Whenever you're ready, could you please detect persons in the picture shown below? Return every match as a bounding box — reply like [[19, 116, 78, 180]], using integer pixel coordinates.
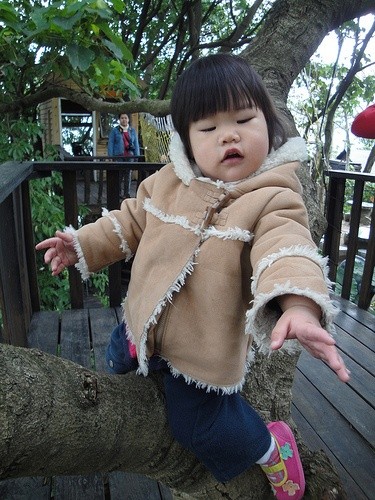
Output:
[[35, 53, 351, 500], [108, 113, 140, 199]]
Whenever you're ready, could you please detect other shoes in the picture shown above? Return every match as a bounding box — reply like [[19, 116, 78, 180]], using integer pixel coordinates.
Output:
[[260, 421, 305, 500]]
